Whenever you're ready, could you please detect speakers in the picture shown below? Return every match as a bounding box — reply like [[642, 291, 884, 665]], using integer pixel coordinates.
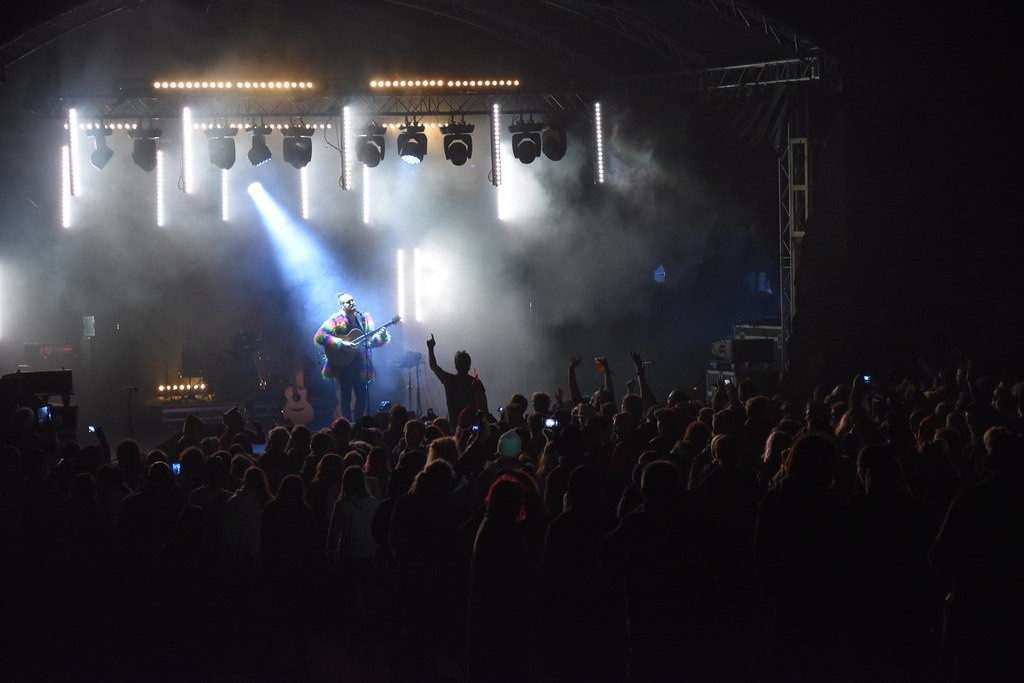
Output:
[[78, 333, 142, 430], [234, 331, 274, 404], [181, 346, 203, 377]]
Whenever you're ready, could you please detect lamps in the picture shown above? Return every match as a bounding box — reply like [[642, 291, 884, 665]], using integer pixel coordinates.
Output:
[[127, 118, 162, 172], [439, 114, 475, 166], [281, 115, 315, 170], [111, 79, 128, 96], [203, 116, 238, 170], [86, 118, 114, 171], [509, 110, 567, 164], [246, 117, 272, 166], [353, 116, 387, 167], [397, 114, 428, 164]]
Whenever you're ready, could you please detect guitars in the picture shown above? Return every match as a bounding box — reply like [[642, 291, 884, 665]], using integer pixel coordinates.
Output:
[[281, 346, 315, 425], [324, 314, 402, 368]]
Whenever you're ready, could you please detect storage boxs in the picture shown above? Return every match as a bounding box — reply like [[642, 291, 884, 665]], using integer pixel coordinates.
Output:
[[706, 370, 735, 405]]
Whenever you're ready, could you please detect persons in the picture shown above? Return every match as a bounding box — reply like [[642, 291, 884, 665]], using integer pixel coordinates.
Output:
[[0, 333, 1024, 682], [313, 293, 391, 422]]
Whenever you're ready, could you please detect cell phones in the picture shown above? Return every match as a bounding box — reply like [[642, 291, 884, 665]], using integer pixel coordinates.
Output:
[[88, 426, 97, 433], [864, 375, 872, 384], [542, 418, 559, 429], [472, 425, 480, 433], [172, 464, 181, 475]]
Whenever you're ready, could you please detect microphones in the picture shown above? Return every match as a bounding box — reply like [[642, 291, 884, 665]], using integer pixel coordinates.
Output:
[[353, 307, 364, 316]]
